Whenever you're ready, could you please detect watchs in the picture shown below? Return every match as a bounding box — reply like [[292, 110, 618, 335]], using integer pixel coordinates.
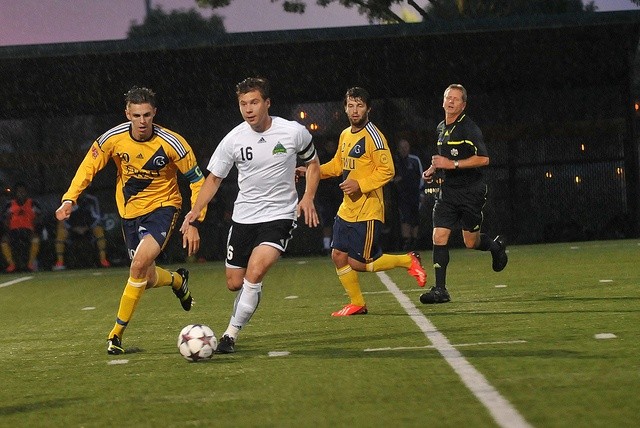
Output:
[[455, 159, 460, 170]]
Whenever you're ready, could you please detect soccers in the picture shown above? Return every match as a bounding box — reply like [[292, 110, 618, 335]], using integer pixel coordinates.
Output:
[[177, 322, 218, 363]]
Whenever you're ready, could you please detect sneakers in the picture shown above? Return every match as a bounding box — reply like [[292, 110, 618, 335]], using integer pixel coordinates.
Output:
[[331, 303, 367, 316], [406, 251, 427, 287], [491, 233, 508, 272], [172, 267, 193, 311], [419, 285, 451, 304], [105, 333, 126, 355], [218, 334, 236, 353]]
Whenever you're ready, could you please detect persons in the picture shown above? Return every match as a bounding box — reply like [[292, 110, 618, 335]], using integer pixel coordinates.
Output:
[[319, 139, 344, 257], [51, 189, 112, 271], [296, 86, 427, 317], [179, 76, 321, 357], [0, 181, 48, 274], [420, 82, 508, 305], [57, 85, 208, 358], [394, 139, 424, 251]]
